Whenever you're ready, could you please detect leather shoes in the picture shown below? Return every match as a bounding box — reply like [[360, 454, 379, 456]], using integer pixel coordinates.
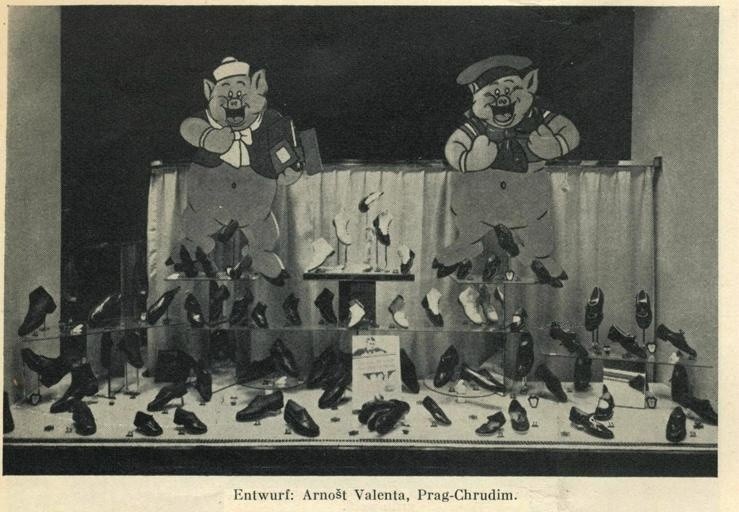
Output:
[[332, 191, 394, 247]]
